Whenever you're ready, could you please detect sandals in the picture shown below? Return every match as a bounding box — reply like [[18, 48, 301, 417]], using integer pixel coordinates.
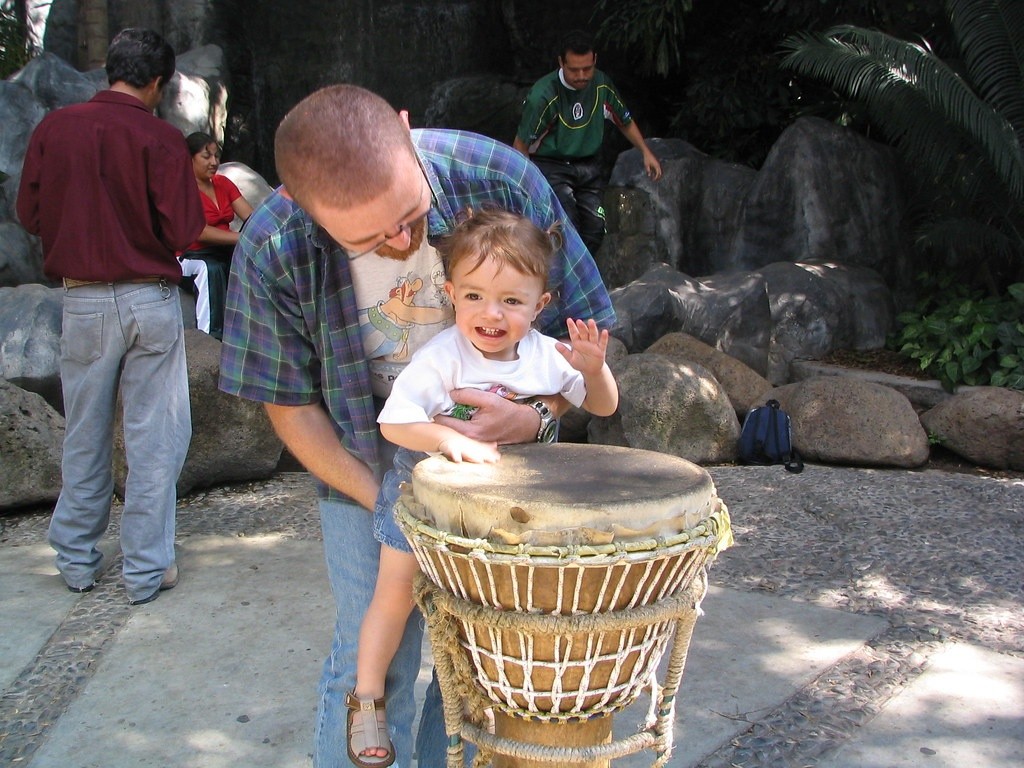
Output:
[[346, 687, 396, 768]]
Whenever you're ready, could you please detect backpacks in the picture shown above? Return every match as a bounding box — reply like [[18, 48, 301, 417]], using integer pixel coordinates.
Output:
[[737, 399, 804, 473]]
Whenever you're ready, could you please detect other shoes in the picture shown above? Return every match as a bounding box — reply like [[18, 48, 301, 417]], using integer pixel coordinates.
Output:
[[132, 563, 180, 607]]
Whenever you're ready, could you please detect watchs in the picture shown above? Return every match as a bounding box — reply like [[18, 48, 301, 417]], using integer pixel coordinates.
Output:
[[523, 397, 558, 444]]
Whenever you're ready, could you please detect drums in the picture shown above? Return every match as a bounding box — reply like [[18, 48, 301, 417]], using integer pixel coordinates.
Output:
[[386, 440, 746, 768]]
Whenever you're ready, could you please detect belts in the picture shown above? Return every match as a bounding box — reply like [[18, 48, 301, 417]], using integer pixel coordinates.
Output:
[[62, 274, 166, 290]]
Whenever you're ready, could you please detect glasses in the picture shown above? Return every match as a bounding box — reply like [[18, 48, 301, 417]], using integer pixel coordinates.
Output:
[[324, 151, 440, 262]]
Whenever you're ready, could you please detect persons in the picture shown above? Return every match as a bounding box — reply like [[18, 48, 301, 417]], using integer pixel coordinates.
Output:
[[168, 131, 255, 342], [14, 27, 209, 607], [215, 82, 619, 767], [512, 28, 662, 259]]
